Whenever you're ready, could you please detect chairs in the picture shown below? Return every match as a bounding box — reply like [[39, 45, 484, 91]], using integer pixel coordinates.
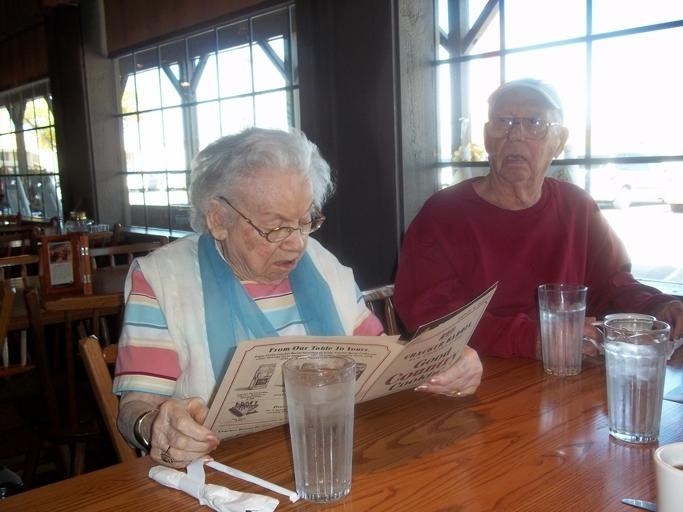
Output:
[[1, 210, 406, 463]]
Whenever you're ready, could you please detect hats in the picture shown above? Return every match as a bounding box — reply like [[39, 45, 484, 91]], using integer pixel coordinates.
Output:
[[489, 77, 560, 111]]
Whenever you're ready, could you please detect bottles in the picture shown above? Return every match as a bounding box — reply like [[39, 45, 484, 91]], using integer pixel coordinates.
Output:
[[63, 216, 93, 236]]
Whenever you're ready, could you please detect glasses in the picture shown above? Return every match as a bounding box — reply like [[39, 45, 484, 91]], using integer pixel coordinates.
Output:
[[219, 196, 326, 244], [488, 118, 560, 139]]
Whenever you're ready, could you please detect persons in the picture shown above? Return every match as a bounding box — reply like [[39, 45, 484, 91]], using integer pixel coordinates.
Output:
[[392, 78, 682, 362], [112, 126, 484, 471]]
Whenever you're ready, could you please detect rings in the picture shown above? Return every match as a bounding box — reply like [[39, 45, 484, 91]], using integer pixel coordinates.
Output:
[[452, 390, 462, 399], [160, 445, 173, 463]]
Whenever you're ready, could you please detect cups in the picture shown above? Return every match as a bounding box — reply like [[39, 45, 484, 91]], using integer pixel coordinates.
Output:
[[537, 283, 683, 512], [283, 354, 357, 503]]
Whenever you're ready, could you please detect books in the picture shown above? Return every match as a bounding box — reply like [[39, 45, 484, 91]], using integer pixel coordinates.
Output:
[[202, 279, 500, 443]]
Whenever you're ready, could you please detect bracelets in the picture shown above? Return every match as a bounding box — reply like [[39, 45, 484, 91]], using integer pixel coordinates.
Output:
[[132, 409, 159, 451]]
